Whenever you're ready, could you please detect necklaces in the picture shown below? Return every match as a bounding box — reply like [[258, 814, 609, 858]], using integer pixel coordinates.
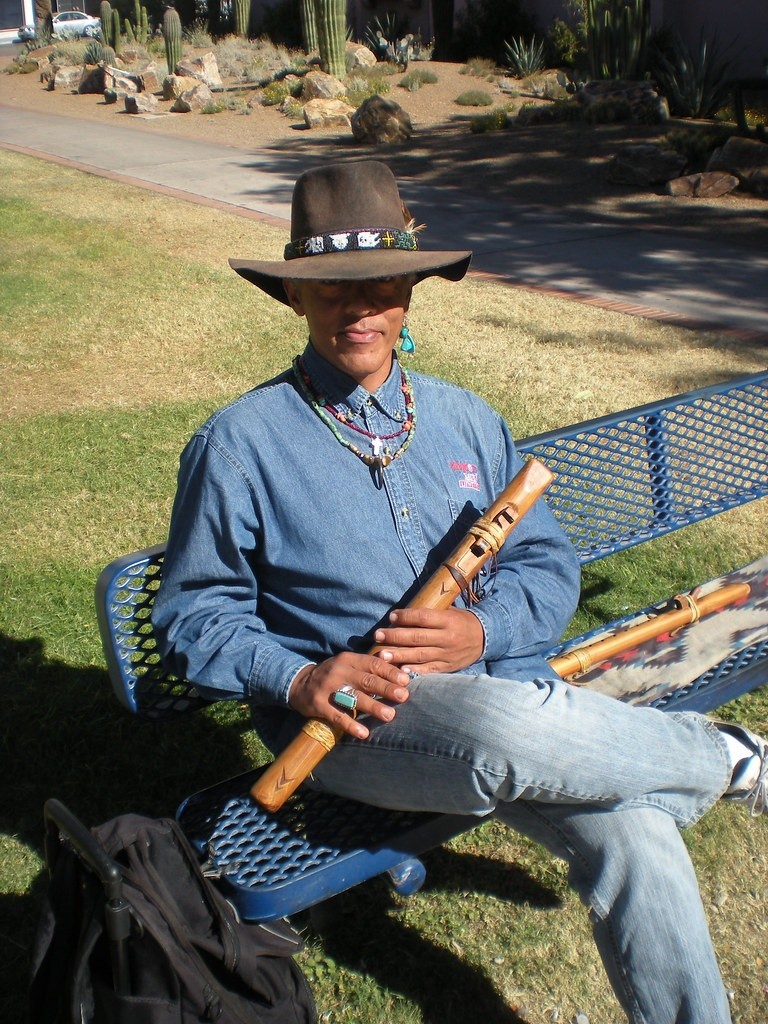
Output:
[[299, 354, 413, 456], [292, 354, 417, 487]]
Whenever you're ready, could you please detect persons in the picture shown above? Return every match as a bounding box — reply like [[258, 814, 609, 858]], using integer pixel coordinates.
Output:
[[151, 162, 768, 1024]]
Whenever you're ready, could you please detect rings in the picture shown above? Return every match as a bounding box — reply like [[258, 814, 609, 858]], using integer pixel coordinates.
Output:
[[332, 685, 357, 710]]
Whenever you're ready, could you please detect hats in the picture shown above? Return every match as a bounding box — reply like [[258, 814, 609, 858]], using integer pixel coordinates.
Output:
[[227, 160, 473, 308]]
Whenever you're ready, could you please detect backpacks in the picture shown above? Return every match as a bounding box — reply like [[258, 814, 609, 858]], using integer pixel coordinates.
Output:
[[24, 796, 318, 1024]]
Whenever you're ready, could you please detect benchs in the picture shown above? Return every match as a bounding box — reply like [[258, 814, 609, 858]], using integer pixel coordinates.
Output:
[[93, 371, 768, 930]]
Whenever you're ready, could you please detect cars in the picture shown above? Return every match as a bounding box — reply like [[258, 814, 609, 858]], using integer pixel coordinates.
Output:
[[17, 10, 101, 41]]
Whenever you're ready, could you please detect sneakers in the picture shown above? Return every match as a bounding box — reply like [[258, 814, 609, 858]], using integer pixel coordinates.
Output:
[[711, 719, 768, 818]]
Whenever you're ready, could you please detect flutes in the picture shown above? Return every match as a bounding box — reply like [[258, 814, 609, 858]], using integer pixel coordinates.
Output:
[[546, 583, 751, 679], [248, 458, 555, 814]]
